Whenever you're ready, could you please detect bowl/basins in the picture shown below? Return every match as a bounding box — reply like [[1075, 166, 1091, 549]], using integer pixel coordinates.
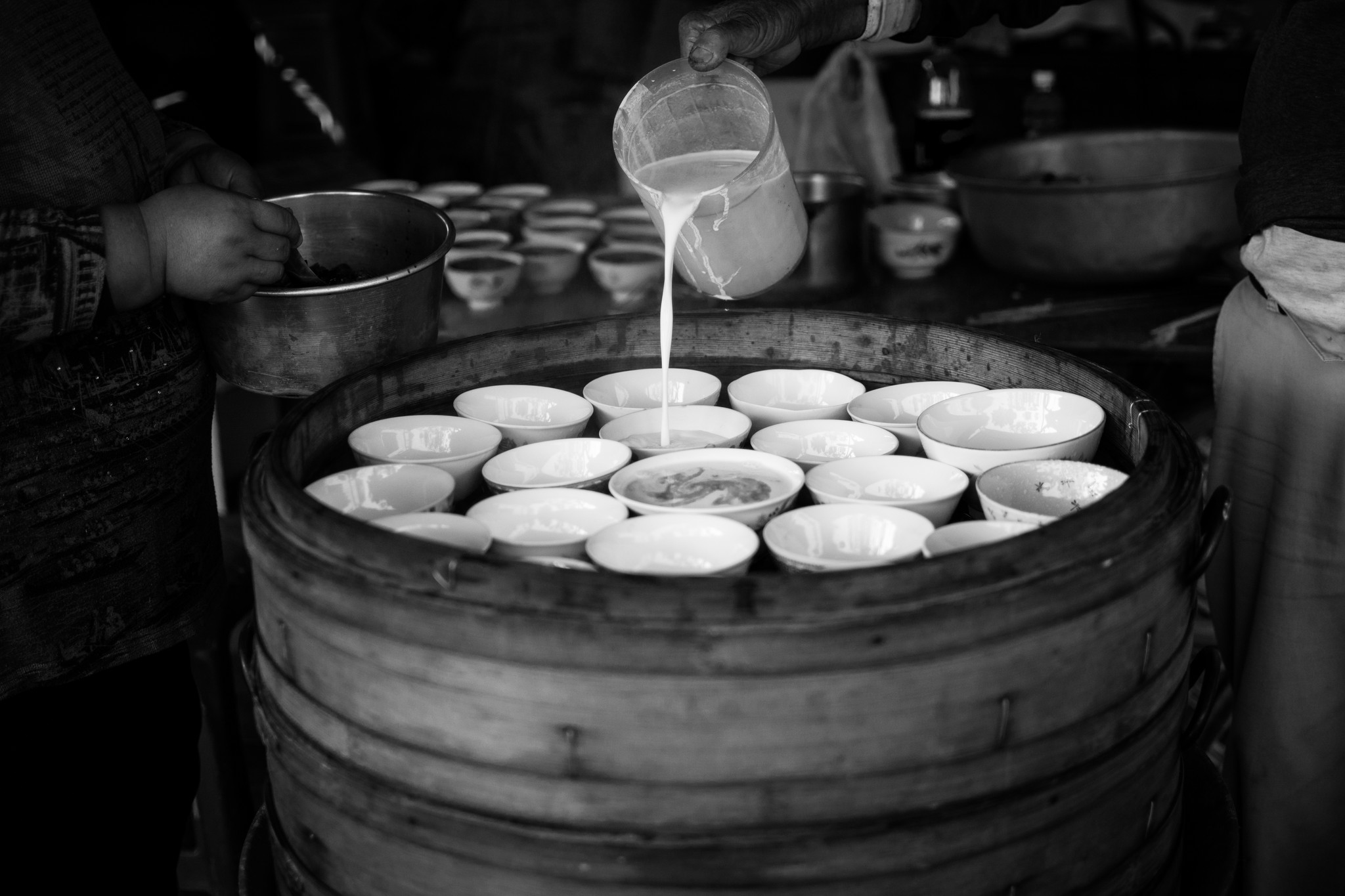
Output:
[[742, 165, 873, 296], [349, 161, 676, 316], [220, 175, 455, 401], [869, 199, 959, 279], [305, 355, 1127, 587], [948, 117, 1245, 279]]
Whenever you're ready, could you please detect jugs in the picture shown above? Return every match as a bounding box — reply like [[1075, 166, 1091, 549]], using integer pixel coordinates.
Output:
[[612, 52, 812, 304]]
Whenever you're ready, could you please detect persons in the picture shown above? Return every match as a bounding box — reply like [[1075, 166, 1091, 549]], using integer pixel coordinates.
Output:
[[678, 0, 1345, 895], [2, 3, 309, 896]]
